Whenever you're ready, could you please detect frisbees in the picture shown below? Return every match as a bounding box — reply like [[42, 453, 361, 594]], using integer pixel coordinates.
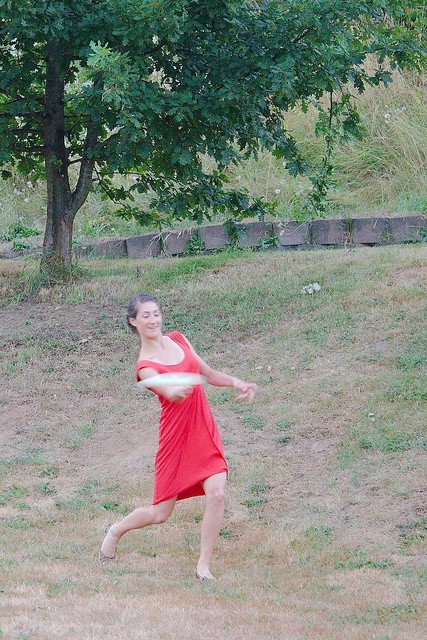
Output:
[[138, 373, 206, 387]]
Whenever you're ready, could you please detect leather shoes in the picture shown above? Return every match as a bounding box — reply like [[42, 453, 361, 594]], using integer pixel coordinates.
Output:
[[195, 565, 217, 581], [99, 546, 116, 566]]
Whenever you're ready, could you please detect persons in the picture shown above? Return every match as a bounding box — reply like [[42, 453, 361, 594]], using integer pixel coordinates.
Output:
[[100, 294, 257, 581]]
